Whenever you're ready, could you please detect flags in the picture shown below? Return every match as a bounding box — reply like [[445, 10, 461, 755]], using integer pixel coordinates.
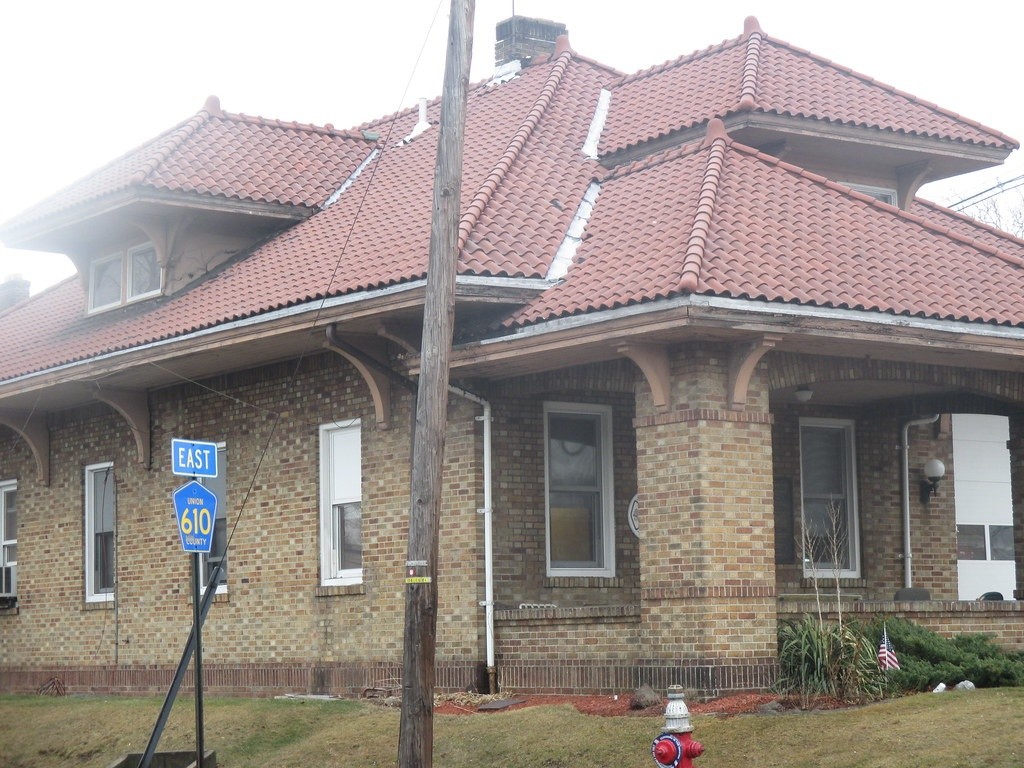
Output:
[[878, 627, 900, 672]]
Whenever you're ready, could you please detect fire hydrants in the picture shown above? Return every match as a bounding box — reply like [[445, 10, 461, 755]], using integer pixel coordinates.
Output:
[[653, 683, 704, 768]]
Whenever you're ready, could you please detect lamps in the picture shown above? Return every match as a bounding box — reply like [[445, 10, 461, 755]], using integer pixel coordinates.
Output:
[[920, 459, 945, 503], [795, 388, 814, 402]]
